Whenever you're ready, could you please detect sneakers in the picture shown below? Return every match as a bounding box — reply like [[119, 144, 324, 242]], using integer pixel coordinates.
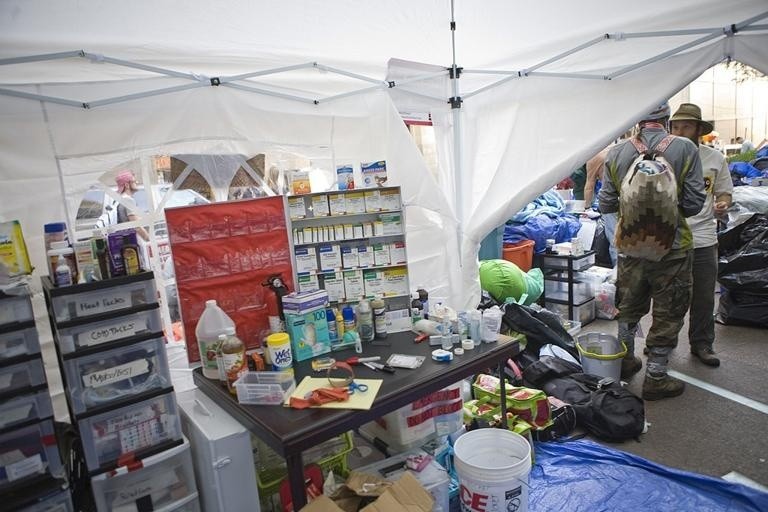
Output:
[[691, 342, 720, 366], [643, 374, 683, 400], [621, 356, 641, 377]]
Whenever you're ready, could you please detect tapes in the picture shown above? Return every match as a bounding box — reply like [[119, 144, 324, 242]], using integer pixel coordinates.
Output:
[[327, 362, 353, 387]]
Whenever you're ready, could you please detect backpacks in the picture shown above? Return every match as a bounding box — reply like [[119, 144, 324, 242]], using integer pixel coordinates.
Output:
[[615, 137, 678, 262]]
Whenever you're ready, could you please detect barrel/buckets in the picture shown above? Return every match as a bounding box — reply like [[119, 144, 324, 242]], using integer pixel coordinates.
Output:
[[195, 299, 237, 381], [453, 427, 532, 511]]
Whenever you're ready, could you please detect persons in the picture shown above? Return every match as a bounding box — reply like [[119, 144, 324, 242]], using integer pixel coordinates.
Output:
[[583, 141, 620, 268], [735, 136, 755, 155], [667, 102, 734, 368], [597, 100, 707, 402], [111, 169, 150, 244]]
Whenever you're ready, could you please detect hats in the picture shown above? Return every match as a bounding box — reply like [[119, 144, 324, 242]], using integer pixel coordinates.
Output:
[[670, 104, 713, 135], [115, 172, 134, 193], [644, 102, 670, 121]]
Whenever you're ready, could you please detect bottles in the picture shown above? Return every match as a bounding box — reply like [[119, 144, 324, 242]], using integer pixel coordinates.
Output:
[[291, 171, 311, 194], [410, 284, 429, 327], [326, 295, 387, 344], [43, 222, 78, 286], [337, 163, 354, 190], [442, 309, 501, 350], [214, 327, 249, 394], [96, 239, 115, 280], [267, 333, 293, 368], [120, 234, 141, 276]]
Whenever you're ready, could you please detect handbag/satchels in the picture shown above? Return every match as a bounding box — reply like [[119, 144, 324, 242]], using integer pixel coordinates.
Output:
[[463, 303, 644, 465]]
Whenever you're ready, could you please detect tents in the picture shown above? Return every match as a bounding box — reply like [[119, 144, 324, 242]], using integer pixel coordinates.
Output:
[[1, 1, 768, 511]]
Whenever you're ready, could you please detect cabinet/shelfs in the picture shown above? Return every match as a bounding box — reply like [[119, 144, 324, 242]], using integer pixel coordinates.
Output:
[[536, 250, 597, 328], [191, 329, 521, 512], [0, 274, 74, 512], [287, 186, 414, 335], [40, 269, 203, 512]]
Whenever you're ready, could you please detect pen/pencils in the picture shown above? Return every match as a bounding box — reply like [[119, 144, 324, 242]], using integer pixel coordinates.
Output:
[[363, 362, 375, 370], [346, 356, 381, 364], [368, 361, 395, 373]]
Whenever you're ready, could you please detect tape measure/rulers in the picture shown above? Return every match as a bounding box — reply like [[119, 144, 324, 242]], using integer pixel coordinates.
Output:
[[431, 349, 453, 362]]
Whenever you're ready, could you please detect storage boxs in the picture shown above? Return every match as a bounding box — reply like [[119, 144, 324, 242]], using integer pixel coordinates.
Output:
[[351, 447, 449, 512], [298, 471, 434, 511], [503, 239, 535, 273]]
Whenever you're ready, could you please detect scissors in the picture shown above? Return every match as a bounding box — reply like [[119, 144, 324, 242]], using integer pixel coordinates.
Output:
[[346, 380, 369, 395]]
